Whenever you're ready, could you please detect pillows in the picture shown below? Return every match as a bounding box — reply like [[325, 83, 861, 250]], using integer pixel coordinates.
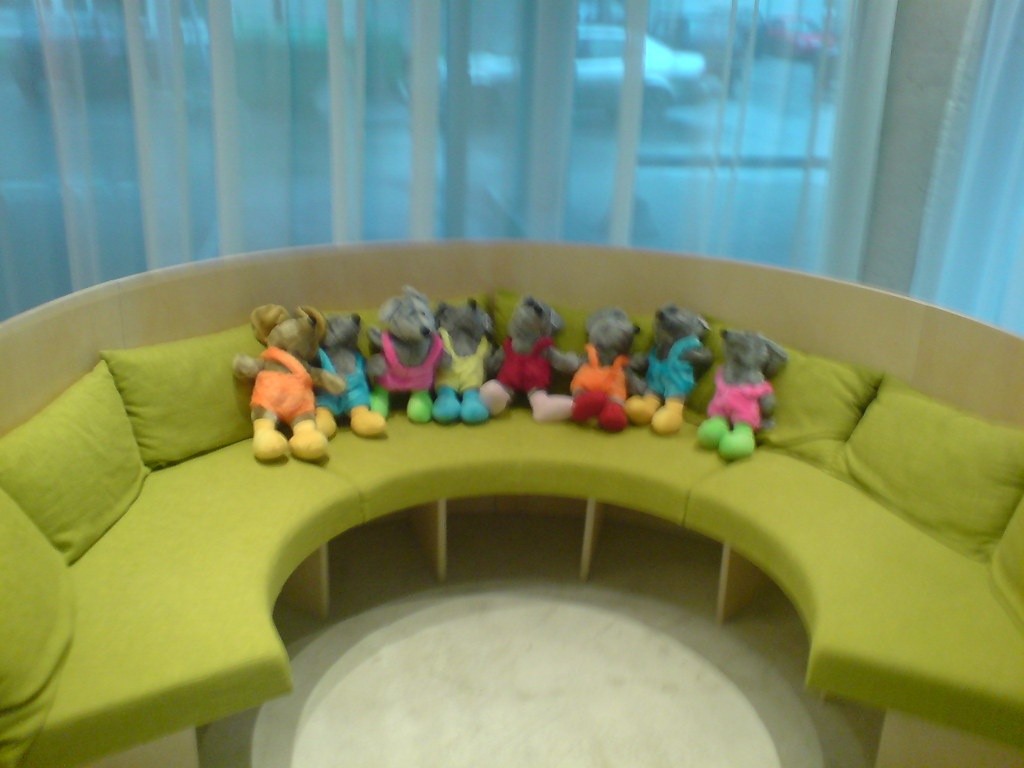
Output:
[[322, 291, 491, 387], [494, 285, 661, 392], [100, 322, 265, 470], [1, 360, 152, 565], [0, 487, 76, 768], [687, 320, 884, 465], [841, 373, 1022, 558]]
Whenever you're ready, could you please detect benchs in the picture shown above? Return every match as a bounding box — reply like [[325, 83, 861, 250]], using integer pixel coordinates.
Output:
[[0, 239, 1023, 768]]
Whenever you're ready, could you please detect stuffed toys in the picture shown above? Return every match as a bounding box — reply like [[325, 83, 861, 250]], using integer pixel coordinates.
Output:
[[571, 307, 646, 432], [623, 305, 715, 432], [369, 286, 497, 423], [697, 327, 787, 460], [480, 296, 580, 421], [234, 304, 388, 459]]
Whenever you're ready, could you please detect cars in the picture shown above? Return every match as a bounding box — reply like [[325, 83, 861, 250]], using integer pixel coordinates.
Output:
[[747, 12, 833, 58], [404, 9, 744, 127]]
